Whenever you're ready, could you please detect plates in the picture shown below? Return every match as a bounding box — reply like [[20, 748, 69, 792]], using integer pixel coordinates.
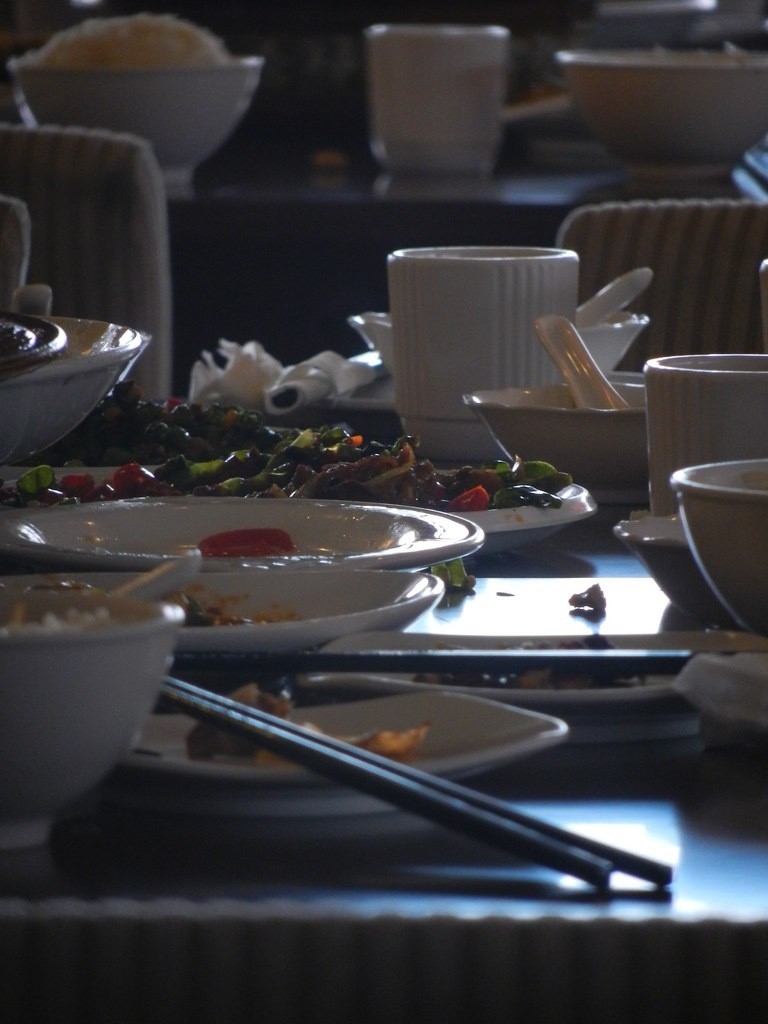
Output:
[[299, 631, 768, 719], [0, 498, 484, 570], [268, 366, 645, 435], [0, 571, 446, 657], [0, 466, 598, 558], [100, 691, 568, 816]]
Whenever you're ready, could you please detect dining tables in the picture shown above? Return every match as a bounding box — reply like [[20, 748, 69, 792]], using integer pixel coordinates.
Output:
[[0, 378, 768, 1024]]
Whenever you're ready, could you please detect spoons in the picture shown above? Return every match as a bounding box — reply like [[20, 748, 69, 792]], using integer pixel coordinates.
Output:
[[536, 314, 630, 408]]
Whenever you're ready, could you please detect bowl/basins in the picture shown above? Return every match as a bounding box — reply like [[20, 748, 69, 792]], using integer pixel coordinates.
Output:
[[0, 590, 185, 849], [671, 459, 768, 638], [466, 383, 651, 505], [0, 315, 150, 465], [7, 55, 264, 188], [347, 312, 649, 377], [614, 514, 737, 628], [556, 49, 768, 176]]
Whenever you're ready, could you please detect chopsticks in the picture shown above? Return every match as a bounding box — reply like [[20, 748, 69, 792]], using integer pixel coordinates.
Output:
[[163, 675, 672, 885], [171, 648, 690, 675]]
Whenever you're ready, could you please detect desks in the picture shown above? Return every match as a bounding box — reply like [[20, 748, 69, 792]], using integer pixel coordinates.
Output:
[[174, 165, 768, 378]]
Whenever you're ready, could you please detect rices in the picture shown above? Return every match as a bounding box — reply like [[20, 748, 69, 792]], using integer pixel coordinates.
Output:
[[15, 11, 241, 70]]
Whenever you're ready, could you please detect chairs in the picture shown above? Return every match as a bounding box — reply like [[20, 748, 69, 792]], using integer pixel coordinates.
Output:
[[1, 122, 176, 398], [558, 200, 768, 370]]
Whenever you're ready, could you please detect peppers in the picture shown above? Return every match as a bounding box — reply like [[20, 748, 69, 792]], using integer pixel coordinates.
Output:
[[1, 379, 571, 590]]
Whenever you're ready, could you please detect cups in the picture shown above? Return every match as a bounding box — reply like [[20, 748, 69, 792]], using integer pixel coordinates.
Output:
[[367, 23, 509, 177], [644, 353, 768, 517], [388, 246, 579, 459]]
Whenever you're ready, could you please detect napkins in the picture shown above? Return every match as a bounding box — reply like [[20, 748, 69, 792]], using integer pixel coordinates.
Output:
[[187, 343, 374, 413], [676, 651, 768, 740]]
